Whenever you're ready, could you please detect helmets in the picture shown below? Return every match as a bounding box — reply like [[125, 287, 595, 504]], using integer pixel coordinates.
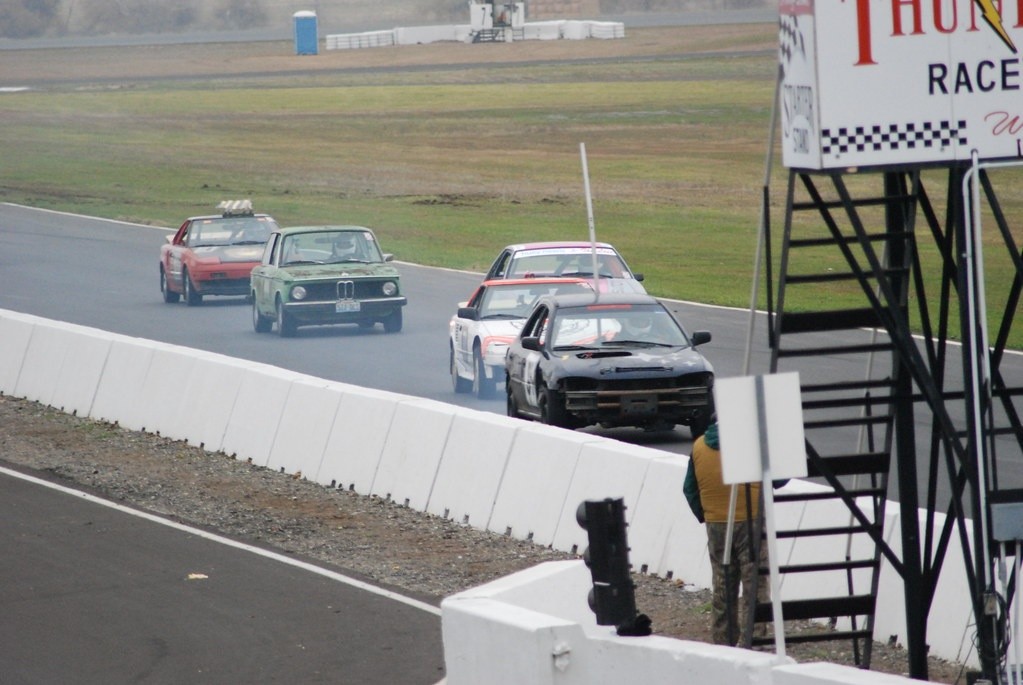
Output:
[[623, 315, 652, 336], [578, 255, 603, 274], [245, 222, 266, 242], [333, 234, 356, 257]]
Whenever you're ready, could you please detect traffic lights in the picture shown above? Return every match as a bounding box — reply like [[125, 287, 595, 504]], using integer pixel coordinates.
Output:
[[576, 496, 639, 628]]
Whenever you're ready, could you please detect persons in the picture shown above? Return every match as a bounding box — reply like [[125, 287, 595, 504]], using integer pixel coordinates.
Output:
[[331, 234, 368, 260], [614, 313, 661, 340], [683, 412, 792, 652], [576, 255, 604, 271]]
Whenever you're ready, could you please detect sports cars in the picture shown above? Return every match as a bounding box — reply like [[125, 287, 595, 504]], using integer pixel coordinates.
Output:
[[484, 238, 650, 309], [450, 276, 619, 402], [159, 199, 282, 308], [250, 224, 407, 338], [502, 290, 716, 438]]
